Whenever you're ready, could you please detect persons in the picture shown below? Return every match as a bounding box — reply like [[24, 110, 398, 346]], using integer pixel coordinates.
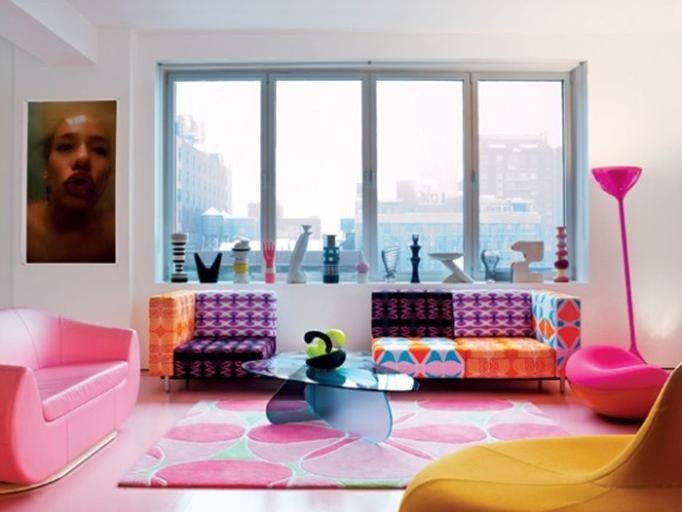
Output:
[[27, 113, 115, 261]]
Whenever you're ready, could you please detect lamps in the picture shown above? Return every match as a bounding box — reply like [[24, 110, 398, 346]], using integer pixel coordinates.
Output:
[[593, 164, 647, 360]]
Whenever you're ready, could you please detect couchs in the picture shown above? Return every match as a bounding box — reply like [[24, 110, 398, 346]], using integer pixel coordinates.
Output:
[[2, 306, 142, 486], [398, 362, 682, 511], [371, 289, 581, 393], [149, 289, 277, 393], [566, 345, 669, 420]]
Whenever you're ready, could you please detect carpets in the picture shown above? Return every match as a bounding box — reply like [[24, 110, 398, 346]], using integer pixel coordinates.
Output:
[[118, 398, 572, 489]]
